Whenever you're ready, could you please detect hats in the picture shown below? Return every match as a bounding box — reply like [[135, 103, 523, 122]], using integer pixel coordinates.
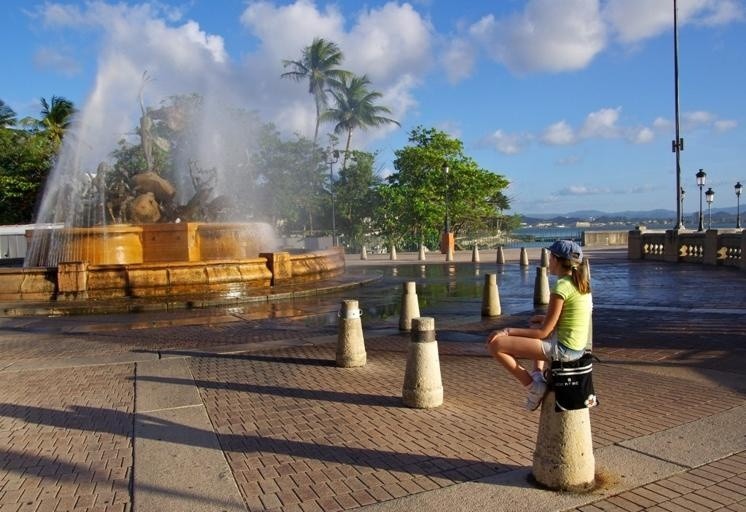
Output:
[[544, 239, 584, 260]]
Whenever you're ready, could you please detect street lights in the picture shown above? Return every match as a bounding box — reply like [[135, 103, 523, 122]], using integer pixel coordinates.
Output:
[[442, 162, 450, 233], [321, 150, 339, 246], [680, 169, 743, 232]]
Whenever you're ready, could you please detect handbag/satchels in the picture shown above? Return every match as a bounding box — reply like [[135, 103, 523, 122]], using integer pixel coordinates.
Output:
[[552, 355, 601, 413]]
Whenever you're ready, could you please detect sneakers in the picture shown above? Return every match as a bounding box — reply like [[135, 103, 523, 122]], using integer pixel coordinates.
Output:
[[532, 371, 546, 384], [526, 383, 547, 411]]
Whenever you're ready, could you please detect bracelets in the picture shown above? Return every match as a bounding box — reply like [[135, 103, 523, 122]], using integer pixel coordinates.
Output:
[[501, 327, 512, 336]]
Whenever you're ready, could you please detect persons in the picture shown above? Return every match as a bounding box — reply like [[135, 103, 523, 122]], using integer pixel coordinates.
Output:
[[136, 89, 162, 173], [485, 239, 593, 411]]
